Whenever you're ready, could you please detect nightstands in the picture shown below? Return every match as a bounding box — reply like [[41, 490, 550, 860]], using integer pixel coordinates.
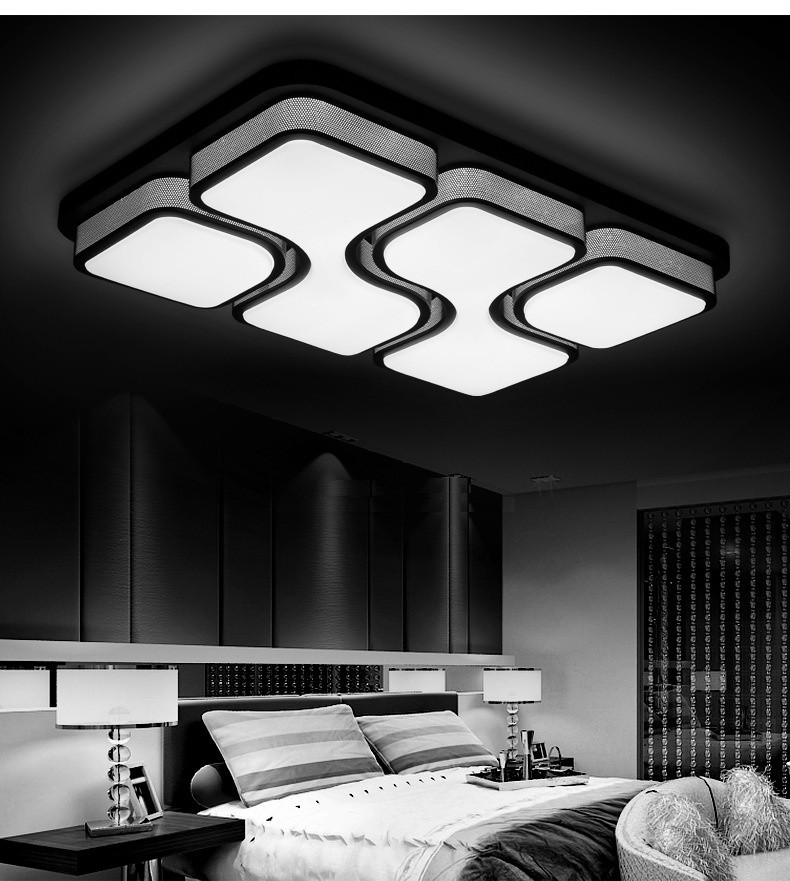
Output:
[[498, 754, 574, 772]]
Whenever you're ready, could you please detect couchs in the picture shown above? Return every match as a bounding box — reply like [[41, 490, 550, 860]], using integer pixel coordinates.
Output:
[[615, 775, 790, 881]]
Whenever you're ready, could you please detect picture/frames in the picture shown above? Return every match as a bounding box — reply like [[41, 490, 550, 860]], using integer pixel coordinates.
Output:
[[124, 762, 164, 821]]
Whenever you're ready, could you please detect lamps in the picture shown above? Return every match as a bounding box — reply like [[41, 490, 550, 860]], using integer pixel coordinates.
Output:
[[56, 30, 730, 397], [56, 669, 179, 837], [483, 667, 542, 760], [388, 667, 446, 691], [691, 671, 727, 692]]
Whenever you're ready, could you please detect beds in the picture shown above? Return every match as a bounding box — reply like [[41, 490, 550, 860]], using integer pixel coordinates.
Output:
[[643, 660, 790, 784], [164, 691, 666, 881]]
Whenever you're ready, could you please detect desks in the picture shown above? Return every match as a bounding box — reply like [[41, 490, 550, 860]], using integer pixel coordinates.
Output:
[[0, 811, 245, 881]]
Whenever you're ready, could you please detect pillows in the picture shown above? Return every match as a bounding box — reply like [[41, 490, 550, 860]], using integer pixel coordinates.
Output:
[[202, 704, 386, 808], [355, 710, 500, 775], [648, 688, 701, 728], [643, 702, 671, 738]]
